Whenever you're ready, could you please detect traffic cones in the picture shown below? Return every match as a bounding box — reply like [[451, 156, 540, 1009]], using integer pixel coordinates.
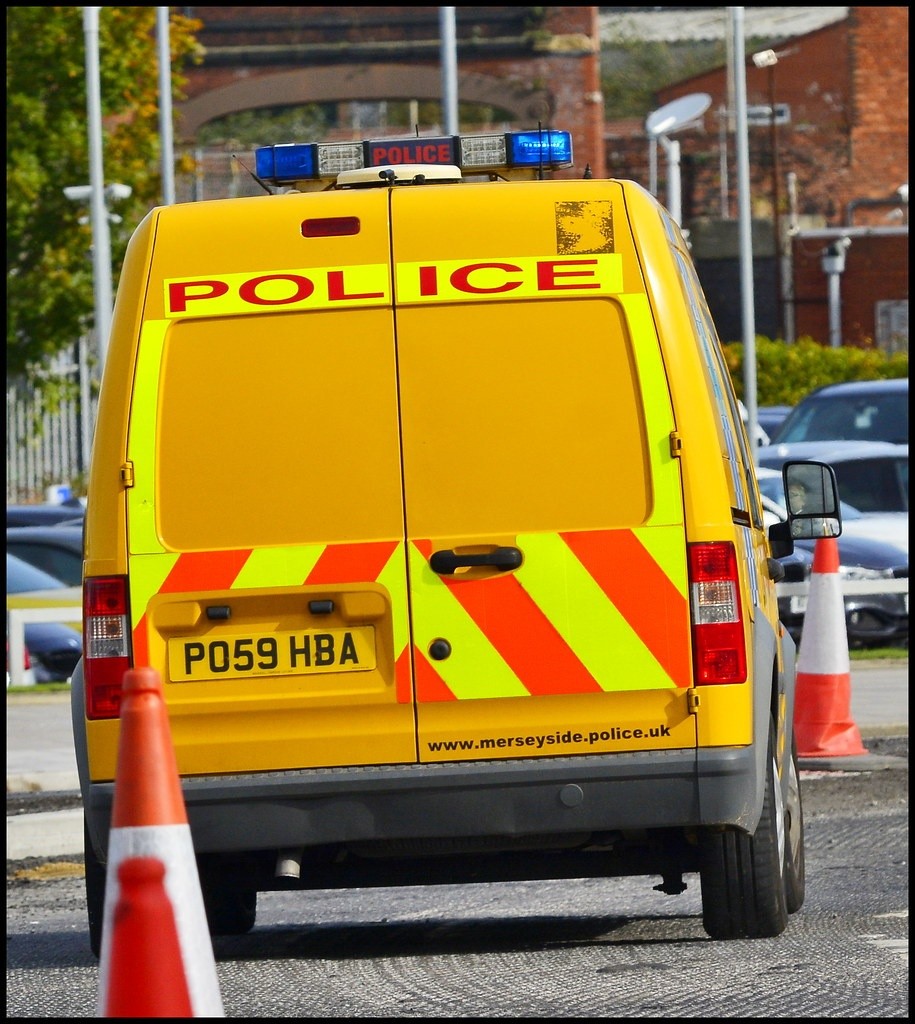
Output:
[[106, 857, 197, 1019], [89, 668, 225, 1017], [792, 537, 874, 763]]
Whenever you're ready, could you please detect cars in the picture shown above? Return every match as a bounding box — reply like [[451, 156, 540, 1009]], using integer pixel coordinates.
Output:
[[6, 503, 86, 681], [736, 375, 910, 648]]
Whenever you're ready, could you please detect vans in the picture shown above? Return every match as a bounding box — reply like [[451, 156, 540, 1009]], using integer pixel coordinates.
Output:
[[75, 129, 841, 951]]
